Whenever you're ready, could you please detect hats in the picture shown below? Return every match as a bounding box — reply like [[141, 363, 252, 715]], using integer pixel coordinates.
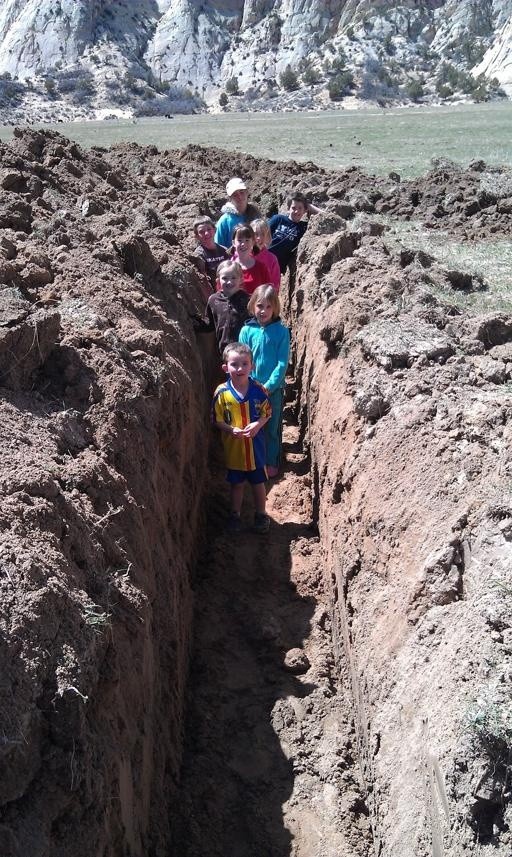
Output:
[[226, 177, 248, 196]]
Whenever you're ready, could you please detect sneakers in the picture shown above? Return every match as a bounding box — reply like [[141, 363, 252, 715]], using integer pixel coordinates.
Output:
[[226, 507, 272, 535], [264, 464, 280, 481]]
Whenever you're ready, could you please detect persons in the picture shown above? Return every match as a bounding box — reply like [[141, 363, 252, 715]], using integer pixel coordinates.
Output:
[[267, 190, 325, 278], [187, 213, 229, 293], [213, 176, 263, 250], [235, 282, 291, 479], [215, 221, 270, 298], [210, 341, 273, 539], [187, 259, 253, 361], [250, 216, 282, 296]]
